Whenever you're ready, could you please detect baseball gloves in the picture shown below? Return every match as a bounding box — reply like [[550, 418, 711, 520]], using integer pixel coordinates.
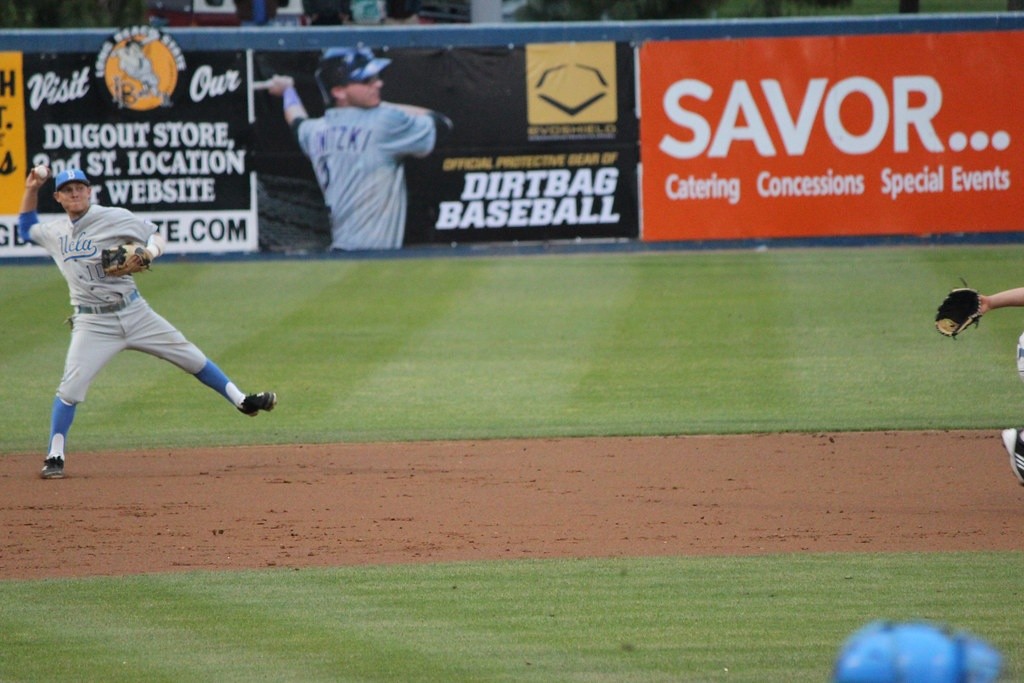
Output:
[[101, 244, 153, 277], [932, 275, 982, 342]]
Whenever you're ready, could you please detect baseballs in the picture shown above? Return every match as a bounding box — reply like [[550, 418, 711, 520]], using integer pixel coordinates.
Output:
[[34, 165, 48, 179]]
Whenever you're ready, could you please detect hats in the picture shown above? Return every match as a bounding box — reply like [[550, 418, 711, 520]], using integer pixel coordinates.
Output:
[[55, 168, 89, 191]]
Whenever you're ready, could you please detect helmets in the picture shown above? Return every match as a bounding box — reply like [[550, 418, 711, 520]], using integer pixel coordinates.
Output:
[[832, 620, 1001, 683], [314, 43, 391, 105]]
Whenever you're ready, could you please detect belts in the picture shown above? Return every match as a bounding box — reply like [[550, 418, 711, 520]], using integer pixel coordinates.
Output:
[[78, 292, 138, 314]]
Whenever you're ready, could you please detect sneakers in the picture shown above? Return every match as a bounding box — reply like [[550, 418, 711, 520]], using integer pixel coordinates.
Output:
[[39, 456, 65, 479], [239, 391, 277, 416], [1000, 427, 1024, 484]]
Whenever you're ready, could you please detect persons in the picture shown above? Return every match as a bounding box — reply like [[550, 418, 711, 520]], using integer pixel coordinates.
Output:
[[936, 286, 1024, 484], [16, 165, 277, 479], [269, 48, 454, 252]]
